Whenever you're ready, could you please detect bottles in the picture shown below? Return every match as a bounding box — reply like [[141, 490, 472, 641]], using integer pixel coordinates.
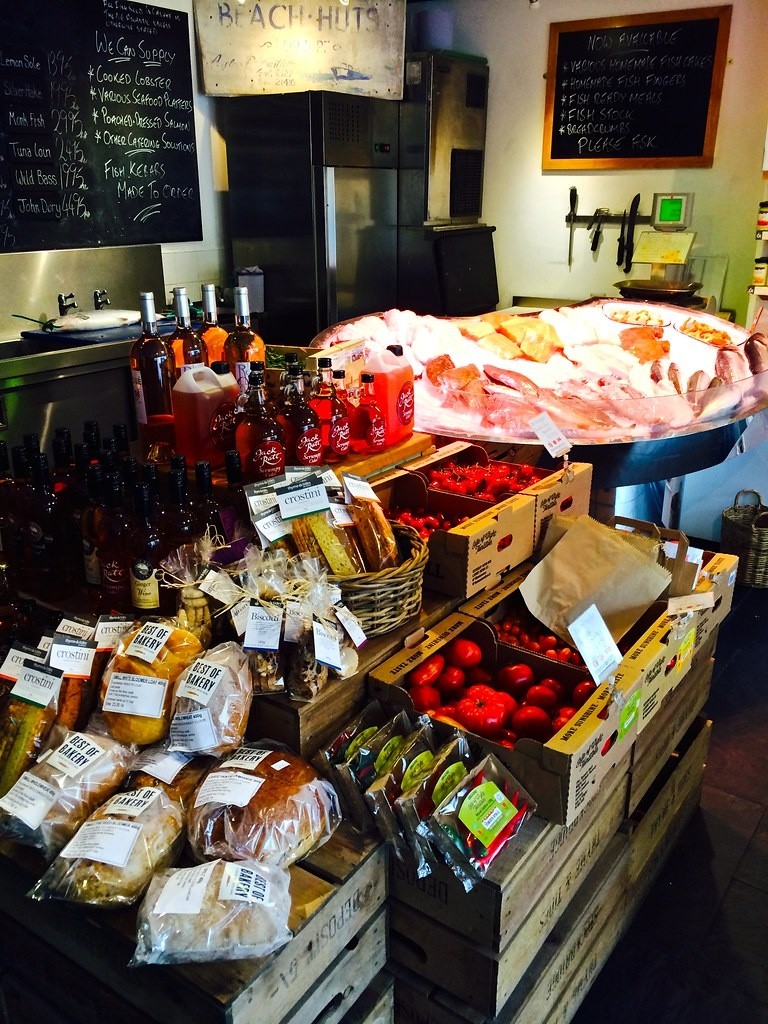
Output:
[[129, 292, 176, 426], [169, 456, 198, 554], [306, 359, 348, 464], [195, 461, 231, 547], [165, 287, 213, 417], [277, 366, 318, 464], [359, 345, 413, 446], [349, 373, 389, 456], [277, 352, 310, 406], [223, 286, 267, 407], [1, 419, 164, 641], [171, 362, 240, 472], [225, 451, 255, 531], [235, 361, 286, 483], [200, 284, 230, 373], [333, 370, 358, 410]]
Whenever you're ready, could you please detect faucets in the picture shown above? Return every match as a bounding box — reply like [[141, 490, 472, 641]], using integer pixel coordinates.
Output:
[[58, 292, 78, 316], [93, 289, 111, 310]]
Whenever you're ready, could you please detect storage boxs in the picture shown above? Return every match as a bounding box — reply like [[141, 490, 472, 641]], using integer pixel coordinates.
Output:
[[626, 656, 716, 823], [0, 820, 386, 1024], [366, 614, 640, 828], [631, 622, 720, 773], [342, 469, 535, 598], [388, 775, 629, 1019], [459, 562, 697, 735], [396, 442, 592, 554], [0, 903, 389, 1024], [665, 542, 740, 658], [388, 747, 633, 954], [394, 891, 631, 1024], [619, 714, 714, 884], [627, 753, 708, 917], [265, 337, 368, 407], [0, 971, 395, 1024], [394, 838, 630, 1024]]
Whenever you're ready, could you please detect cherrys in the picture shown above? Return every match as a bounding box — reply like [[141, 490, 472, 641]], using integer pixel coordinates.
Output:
[[428, 461, 544, 503]]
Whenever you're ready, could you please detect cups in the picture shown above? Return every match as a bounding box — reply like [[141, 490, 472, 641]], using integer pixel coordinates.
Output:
[[665, 264, 686, 291]]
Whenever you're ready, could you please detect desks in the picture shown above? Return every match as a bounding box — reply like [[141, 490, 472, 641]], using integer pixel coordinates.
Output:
[[247, 601, 452, 760]]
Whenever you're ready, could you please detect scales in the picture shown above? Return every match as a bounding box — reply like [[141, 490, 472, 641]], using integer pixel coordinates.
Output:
[[613, 192, 708, 311]]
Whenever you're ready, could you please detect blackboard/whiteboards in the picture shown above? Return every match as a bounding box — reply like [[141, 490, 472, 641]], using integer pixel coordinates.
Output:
[[541, 4, 733, 171], [0, 0, 204, 255]]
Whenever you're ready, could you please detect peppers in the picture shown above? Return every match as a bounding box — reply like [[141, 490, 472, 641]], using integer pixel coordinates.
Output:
[[405, 638, 612, 756]]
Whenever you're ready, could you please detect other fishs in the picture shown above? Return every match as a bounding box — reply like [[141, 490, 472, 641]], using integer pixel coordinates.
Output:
[[432, 332, 768, 435]]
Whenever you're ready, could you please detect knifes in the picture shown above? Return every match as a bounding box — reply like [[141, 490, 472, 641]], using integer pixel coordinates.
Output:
[[624, 193, 641, 272], [616, 210, 626, 266], [567, 188, 578, 266]]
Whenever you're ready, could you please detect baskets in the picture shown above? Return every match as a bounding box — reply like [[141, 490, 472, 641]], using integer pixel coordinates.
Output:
[[288, 518, 430, 639], [720, 489, 768, 589]]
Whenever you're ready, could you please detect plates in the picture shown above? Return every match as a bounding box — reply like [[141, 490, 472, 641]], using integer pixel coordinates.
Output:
[[602, 302, 673, 326], [673, 315, 753, 350], [613, 279, 703, 302]]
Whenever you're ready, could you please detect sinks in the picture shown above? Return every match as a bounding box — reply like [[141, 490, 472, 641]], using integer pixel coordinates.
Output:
[[66, 310, 141, 330]]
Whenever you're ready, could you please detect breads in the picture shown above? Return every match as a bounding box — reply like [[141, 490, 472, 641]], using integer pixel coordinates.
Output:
[[269, 501, 399, 576], [0, 623, 341, 962]]
